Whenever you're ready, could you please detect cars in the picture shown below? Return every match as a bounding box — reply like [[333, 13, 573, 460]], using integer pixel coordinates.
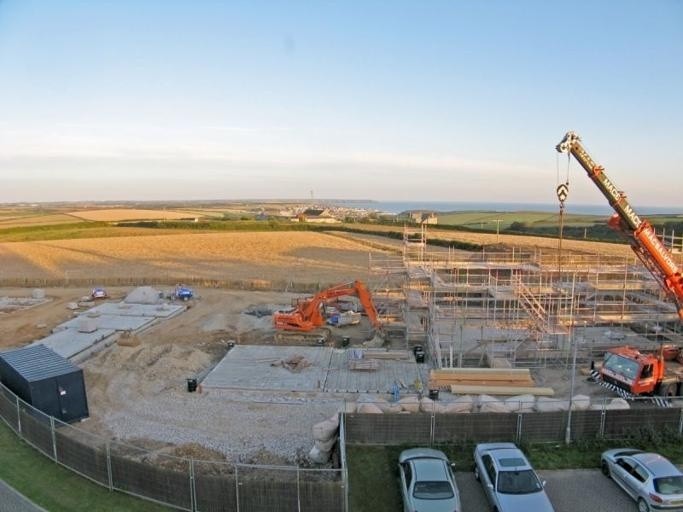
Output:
[[600, 447, 682, 511], [473, 441, 553, 512], [397, 448, 462, 512], [91, 287, 106, 299]]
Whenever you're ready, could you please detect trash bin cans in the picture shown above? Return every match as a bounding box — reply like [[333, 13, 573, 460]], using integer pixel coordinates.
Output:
[[187, 379, 196, 392], [429, 390, 439, 400]]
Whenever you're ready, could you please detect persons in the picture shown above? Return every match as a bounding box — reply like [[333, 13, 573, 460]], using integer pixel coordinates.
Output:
[[413, 376, 425, 400], [392, 378, 404, 405]]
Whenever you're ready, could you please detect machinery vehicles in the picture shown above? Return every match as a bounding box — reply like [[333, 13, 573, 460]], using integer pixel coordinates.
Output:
[[169, 282, 193, 302], [553, 130, 682, 401], [271, 279, 386, 348]]
